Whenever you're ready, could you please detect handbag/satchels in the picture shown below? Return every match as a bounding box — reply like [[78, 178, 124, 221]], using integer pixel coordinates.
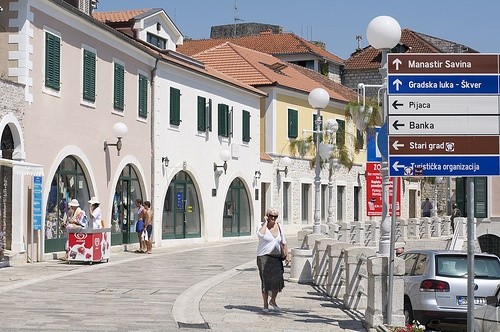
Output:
[[277, 221, 286, 260]]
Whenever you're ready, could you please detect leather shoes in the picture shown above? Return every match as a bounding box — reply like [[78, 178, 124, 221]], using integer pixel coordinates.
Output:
[[263, 308, 268, 314], [269, 300, 281, 311]]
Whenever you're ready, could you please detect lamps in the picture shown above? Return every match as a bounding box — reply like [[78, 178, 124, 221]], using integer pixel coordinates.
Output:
[[214, 150, 231, 175], [357, 163, 366, 179], [162, 157, 169, 168], [104, 122, 128, 156], [278, 157, 292, 176]]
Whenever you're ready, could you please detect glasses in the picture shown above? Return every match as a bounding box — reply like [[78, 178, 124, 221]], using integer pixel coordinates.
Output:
[[269, 214, 278, 218]]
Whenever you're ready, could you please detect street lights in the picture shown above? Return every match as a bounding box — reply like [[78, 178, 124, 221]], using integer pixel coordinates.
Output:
[[349, 15, 402, 257], [324, 119, 339, 223], [302, 88, 334, 234]]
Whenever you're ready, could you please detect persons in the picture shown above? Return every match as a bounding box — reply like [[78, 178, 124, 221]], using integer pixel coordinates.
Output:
[[59, 199, 88, 261], [135, 199, 147, 253], [450, 203, 461, 234], [88, 197, 102, 229], [144, 201, 152, 254], [257, 208, 289, 313], [422, 198, 433, 217]]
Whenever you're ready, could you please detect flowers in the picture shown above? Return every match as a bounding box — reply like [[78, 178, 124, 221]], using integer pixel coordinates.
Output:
[[393, 320, 426, 332]]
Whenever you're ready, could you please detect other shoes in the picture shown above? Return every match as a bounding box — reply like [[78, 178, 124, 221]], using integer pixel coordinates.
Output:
[[135, 248, 153, 254]]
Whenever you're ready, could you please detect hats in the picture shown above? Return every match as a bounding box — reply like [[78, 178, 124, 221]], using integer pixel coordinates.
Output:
[[88, 197, 100, 204], [68, 199, 79, 206]]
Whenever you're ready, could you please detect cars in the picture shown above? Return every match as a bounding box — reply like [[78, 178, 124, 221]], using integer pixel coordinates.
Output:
[[398, 250, 500, 327], [475, 284, 500, 332]]
